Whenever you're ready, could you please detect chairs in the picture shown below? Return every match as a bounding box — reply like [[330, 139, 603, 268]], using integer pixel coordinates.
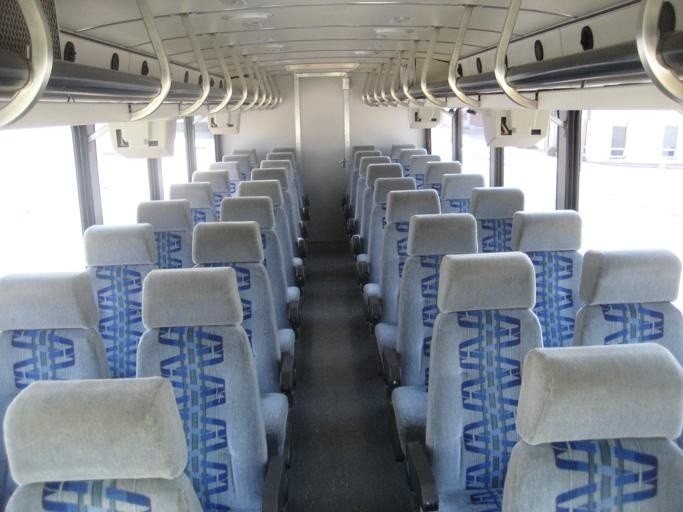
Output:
[[216, 194, 304, 328], [136, 198, 196, 275], [511, 207, 587, 348], [498, 340, 683, 511], [80, 221, 161, 380], [170, 140, 312, 286], [571, 245, 682, 361], [1, 374, 206, 512], [1, 270, 111, 420], [373, 188, 444, 384], [341, 142, 487, 333], [401, 249, 546, 511], [133, 262, 270, 512], [188, 220, 291, 462], [469, 185, 530, 253], [390, 210, 484, 464]]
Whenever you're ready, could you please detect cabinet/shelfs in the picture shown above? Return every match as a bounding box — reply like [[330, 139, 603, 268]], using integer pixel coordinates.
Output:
[[0, 1, 280, 121], [359, 0, 681, 114]]
